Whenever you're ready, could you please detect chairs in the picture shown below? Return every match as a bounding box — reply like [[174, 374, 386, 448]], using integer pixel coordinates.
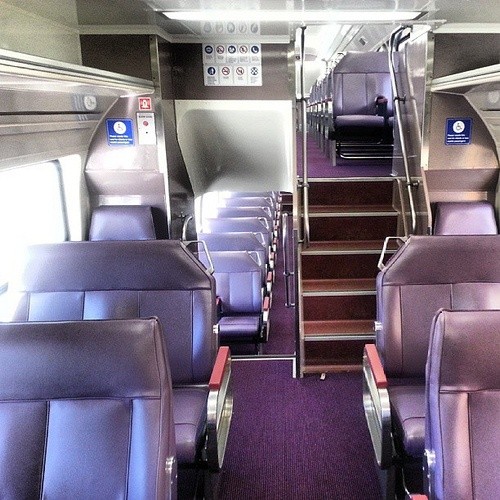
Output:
[[304, 71, 401, 166], [0, 182, 282, 500], [362, 199, 500, 500]]
